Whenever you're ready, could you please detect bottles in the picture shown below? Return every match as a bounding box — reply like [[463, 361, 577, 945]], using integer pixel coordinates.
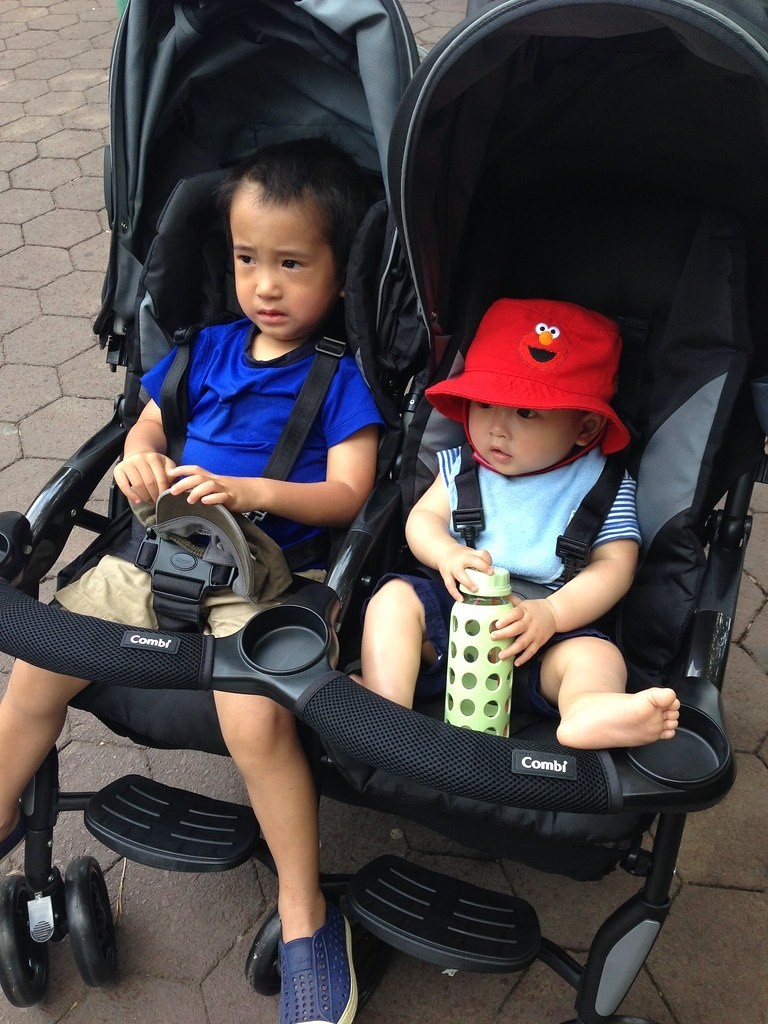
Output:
[[443, 566, 514, 739]]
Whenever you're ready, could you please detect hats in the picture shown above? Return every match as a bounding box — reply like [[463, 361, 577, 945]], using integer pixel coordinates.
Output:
[[422, 298, 631, 455]]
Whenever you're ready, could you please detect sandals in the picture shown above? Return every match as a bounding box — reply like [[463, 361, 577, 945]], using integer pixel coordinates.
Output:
[[278, 900, 359, 1023], [1, 802, 28, 861]]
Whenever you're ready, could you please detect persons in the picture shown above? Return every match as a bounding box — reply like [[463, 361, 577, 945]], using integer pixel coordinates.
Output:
[[349, 299, 681, 749], [0, 137, 386, 1024]]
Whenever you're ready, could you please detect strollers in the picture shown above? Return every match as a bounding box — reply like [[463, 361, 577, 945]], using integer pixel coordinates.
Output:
[[0, 2, 767, 1022]]
